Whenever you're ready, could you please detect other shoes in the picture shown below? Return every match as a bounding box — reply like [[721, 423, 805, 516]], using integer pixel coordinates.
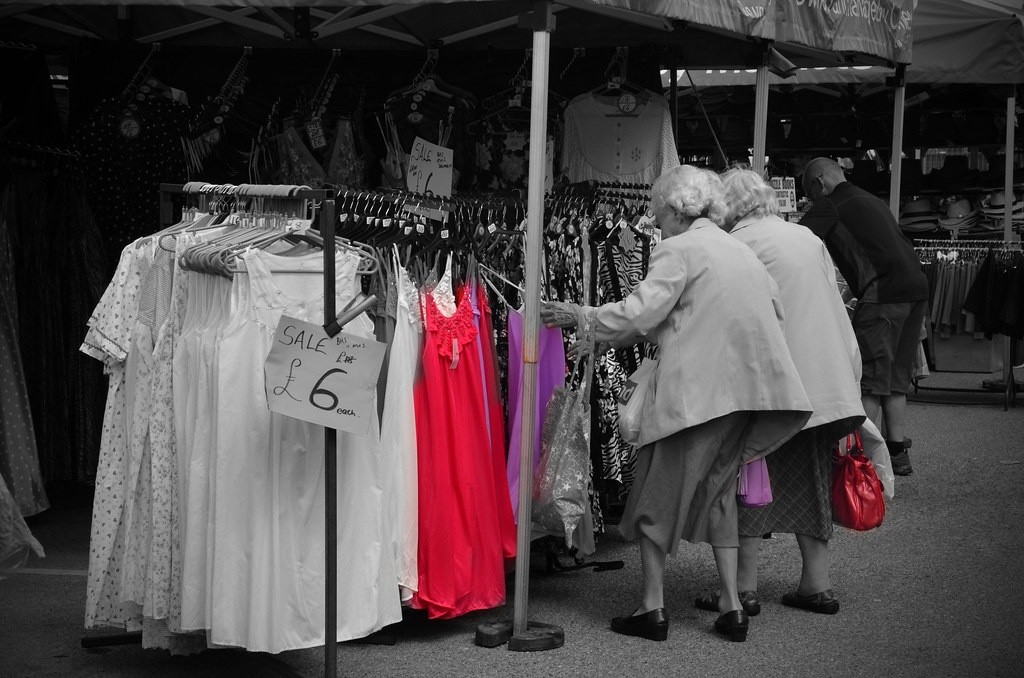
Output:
[[782, 588, 839, 614], [695, 588, 761, 616]]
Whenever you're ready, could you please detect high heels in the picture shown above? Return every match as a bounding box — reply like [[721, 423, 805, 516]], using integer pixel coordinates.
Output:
[[715, 610, 749, 642], [612, 608, 669, 641]]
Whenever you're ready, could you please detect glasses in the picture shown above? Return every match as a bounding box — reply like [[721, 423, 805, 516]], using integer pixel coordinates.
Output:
[[806, 174, 823, 201]]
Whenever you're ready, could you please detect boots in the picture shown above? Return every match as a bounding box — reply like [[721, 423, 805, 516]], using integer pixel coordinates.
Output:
[[885, 437, 913, 475]]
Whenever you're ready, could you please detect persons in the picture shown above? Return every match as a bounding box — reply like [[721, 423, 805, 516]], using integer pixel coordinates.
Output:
[[538, 162, 814, 643], [695, 168, 869, 614], [795, 156, 928, 476]]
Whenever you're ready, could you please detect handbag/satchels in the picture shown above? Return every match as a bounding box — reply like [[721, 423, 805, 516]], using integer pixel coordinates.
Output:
[[618, 349, 659, 446], [839, 418, 895, 501], [532, 307, 597, 550], [830, 428, 886, 531]]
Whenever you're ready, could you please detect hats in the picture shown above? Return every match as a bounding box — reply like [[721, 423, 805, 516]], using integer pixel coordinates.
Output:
[[980, 189, 1024, 231], [898, 200, 941, 233], [938, 199, 979, 230]]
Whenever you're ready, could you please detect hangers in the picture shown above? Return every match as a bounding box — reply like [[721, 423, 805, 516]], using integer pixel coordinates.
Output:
[[0, 139, 83, 162], [120, 42, 653, 268], [916, 239, 1024, 263]]
